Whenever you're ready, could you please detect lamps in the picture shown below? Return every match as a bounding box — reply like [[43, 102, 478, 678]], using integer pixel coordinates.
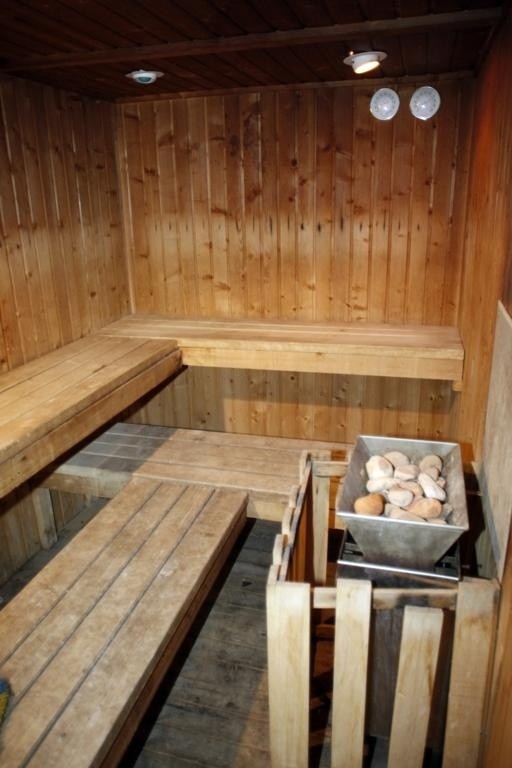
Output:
[[125, 69, 167, 85], [343, 50, 388, 77]]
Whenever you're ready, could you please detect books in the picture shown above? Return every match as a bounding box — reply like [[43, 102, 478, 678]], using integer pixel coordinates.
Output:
[[336, 525, 463, 582]]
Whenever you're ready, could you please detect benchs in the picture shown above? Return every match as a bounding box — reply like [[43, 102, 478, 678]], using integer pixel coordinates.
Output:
[[2, 420, 351, 768], [1, 317, 464, 509]]
